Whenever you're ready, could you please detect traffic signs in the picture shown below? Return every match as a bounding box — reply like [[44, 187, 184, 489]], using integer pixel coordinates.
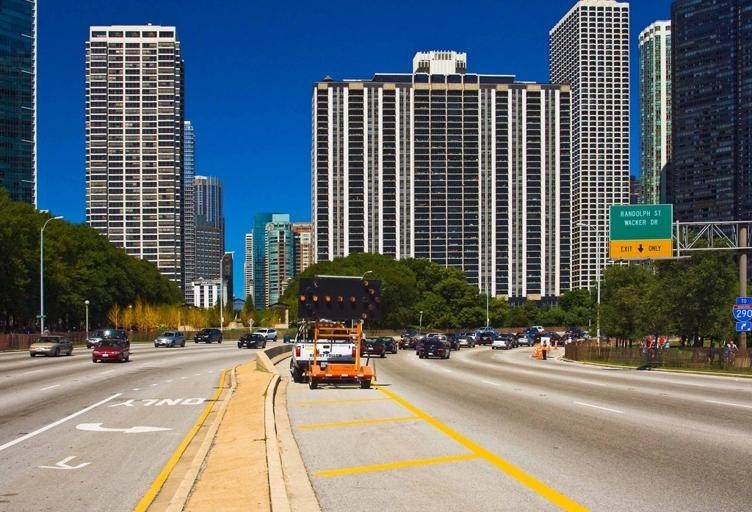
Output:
[[36, 314, 47, 319], [608, 202, 674, 261]]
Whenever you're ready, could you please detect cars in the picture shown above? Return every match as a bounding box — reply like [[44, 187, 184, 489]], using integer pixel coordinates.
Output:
[[477, 326, 494, 333], [252, 328, 278, 342], [361, 334, 386, 359], [397, 330, 591, 350], [379, 335, 399, 354], [28, 334, 74, 357], [91, 337, 132, 364], [529, 325, 545, 333], [237, 334, 268, 349], [153, 329, 186, 349], [412, 335, 451, 360]]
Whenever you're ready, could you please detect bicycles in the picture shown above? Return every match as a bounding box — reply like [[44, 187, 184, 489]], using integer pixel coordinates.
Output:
[[726, 348, 737, 368]]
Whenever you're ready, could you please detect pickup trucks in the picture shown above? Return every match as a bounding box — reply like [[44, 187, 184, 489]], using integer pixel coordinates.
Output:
[[283, 319, 360, 384]]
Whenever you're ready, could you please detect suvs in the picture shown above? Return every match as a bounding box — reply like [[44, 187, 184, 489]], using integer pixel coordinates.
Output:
[[193, 328, 224, 344], [84, 329, 128, 347]]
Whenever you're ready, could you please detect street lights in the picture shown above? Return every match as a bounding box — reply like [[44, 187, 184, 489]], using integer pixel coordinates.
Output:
[[84, 300, 90, 341], [418, 310, 423, 332], [363, 270, 373, 279], [485, 282, 489, 326], [128, 304, 133, 332], [575, 221, 601, 348], [219, 250, 235, 329], [41, 215, 64, 336]]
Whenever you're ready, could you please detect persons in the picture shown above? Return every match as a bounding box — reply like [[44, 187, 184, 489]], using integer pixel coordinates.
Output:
[[658, 336, 665, 348], [726, 340, 738, 365], [664, 341, 669, 349], [646, 336, 652, 348], [542, 339, 547, 360]]
